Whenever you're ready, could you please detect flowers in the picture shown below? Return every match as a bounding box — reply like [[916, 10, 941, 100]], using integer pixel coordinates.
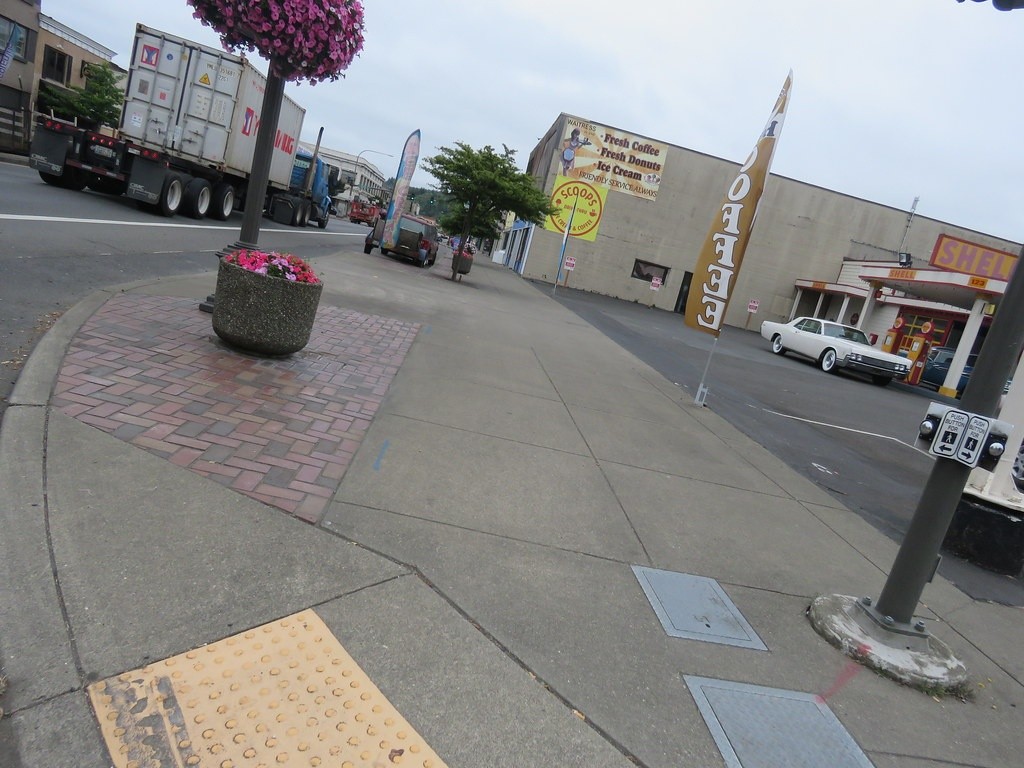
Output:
[[185, 0, 369, 87], [452, 241, 477, 259], [224, 248, 326, 283]]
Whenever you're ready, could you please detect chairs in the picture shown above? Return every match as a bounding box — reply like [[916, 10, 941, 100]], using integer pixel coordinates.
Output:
[[845, 332, 854, 340]]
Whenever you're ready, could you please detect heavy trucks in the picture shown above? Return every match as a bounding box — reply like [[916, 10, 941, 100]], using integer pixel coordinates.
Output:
[[28, 23, 338, 228]]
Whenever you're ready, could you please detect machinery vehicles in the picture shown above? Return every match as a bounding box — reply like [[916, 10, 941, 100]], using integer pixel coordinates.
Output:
[[348, 194, 388, 226]]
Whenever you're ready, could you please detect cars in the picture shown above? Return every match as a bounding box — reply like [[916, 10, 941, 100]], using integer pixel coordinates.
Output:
[[363, 212, 448, 267], [760, 316, 912, 387], [923, 346, 980, 391], [449, 237, 460, 248]]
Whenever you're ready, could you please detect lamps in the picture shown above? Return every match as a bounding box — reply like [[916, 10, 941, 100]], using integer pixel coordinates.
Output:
[[899, 252, 913, 268]]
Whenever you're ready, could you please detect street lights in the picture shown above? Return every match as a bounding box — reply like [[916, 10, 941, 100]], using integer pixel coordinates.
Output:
[[346, 150, 393, 219]]
